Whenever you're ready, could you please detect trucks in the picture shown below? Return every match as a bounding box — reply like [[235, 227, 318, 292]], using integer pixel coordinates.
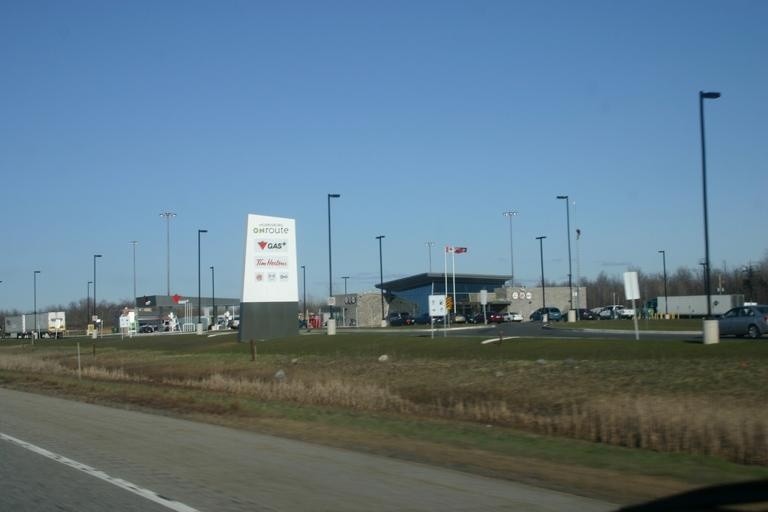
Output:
[[639, 294, 744, 318]]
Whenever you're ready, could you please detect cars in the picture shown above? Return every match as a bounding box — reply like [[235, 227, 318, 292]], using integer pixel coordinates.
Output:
[[231, 316, 241, 333], [573, 308, 600, 321], [713, 303, 768, 338]]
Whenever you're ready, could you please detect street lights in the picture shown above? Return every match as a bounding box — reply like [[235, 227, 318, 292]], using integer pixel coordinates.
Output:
[[698, 88, 725, 318], [657, 248, 670, 320], [33, 211, 218, 339], [699, 261, 708, 294]]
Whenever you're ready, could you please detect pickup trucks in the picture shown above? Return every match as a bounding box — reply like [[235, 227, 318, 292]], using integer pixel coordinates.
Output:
[[596, 304, 637, 320]]
